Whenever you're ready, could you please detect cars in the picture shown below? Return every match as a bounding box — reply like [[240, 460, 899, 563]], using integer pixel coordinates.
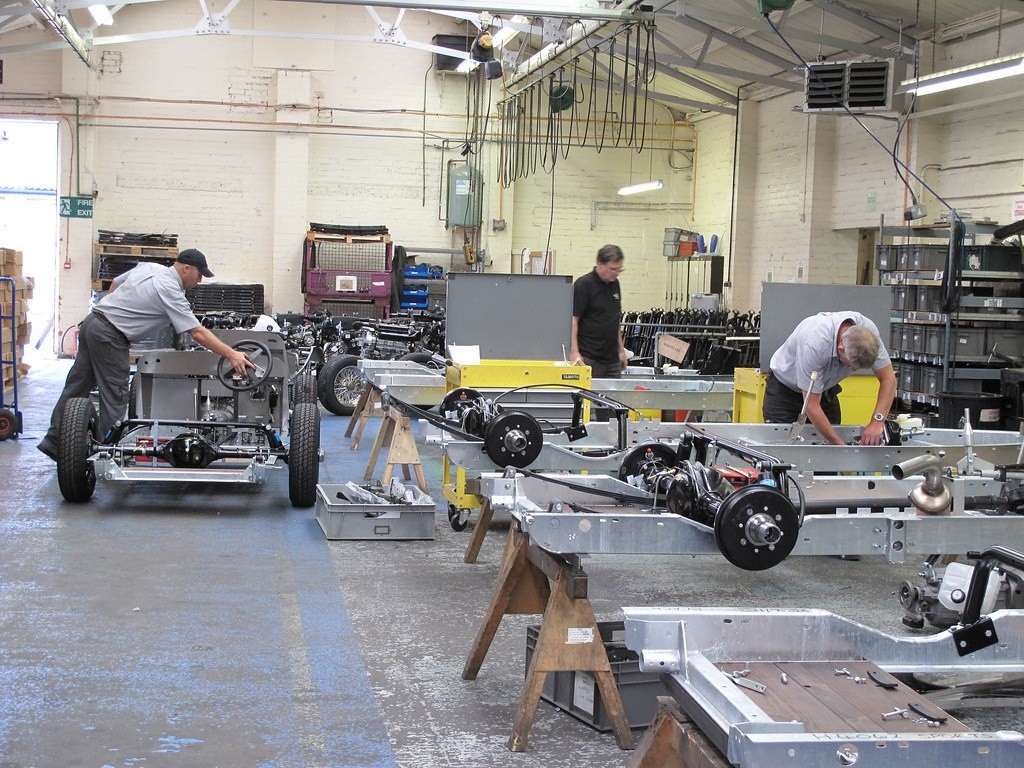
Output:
[[57, 330, 322, 508]]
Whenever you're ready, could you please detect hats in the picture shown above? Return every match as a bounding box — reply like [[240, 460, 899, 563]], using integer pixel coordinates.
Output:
[[177, 248, 214, 277]]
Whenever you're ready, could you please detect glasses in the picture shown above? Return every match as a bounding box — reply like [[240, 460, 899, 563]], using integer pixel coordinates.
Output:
[[605, 264, 626, 275]]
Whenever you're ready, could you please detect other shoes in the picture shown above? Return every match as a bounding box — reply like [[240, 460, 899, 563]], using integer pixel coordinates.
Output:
[[37, 438, 59, 462]]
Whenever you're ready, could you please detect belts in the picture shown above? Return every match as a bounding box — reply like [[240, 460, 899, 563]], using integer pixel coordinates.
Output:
[[94, 312, 129, 343]]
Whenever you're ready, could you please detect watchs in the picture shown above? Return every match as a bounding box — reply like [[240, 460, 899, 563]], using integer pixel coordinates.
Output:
[[872, 413, 886, 422]]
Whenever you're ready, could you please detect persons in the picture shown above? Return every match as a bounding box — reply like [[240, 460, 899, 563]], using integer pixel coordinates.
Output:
[[570, 244, 627, 417], [761, 311, 897, 513], [36, 249, 255, 461]]
